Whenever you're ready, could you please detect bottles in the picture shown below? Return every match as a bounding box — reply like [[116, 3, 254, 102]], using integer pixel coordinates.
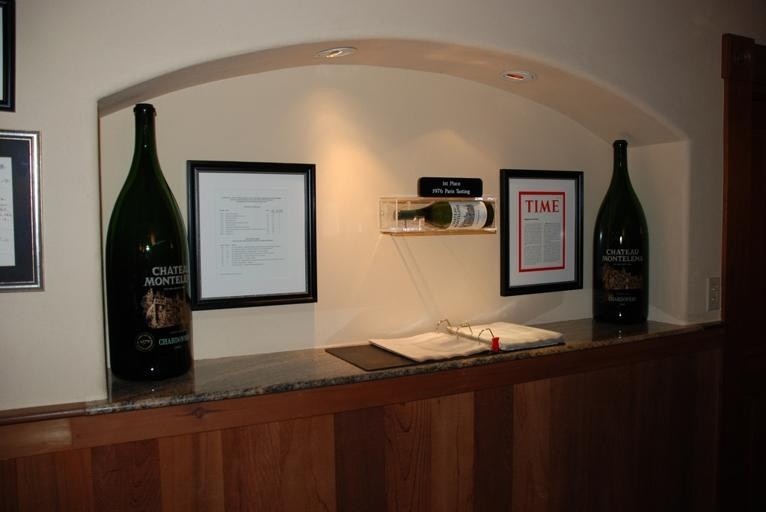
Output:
[[589, 136, 649, 325], [104, 100, 195, 383], [393, 197, 496, 229]]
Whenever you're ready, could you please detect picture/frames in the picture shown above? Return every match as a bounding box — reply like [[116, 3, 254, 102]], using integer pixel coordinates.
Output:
[[499, 168, 584, 296], [0, 0, 15, 112], [0, 130, 41, 290], [186, 159, 317, 311]]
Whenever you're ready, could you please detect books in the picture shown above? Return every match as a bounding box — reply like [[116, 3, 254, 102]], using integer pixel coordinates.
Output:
[[369, 319, 566, 363]]
